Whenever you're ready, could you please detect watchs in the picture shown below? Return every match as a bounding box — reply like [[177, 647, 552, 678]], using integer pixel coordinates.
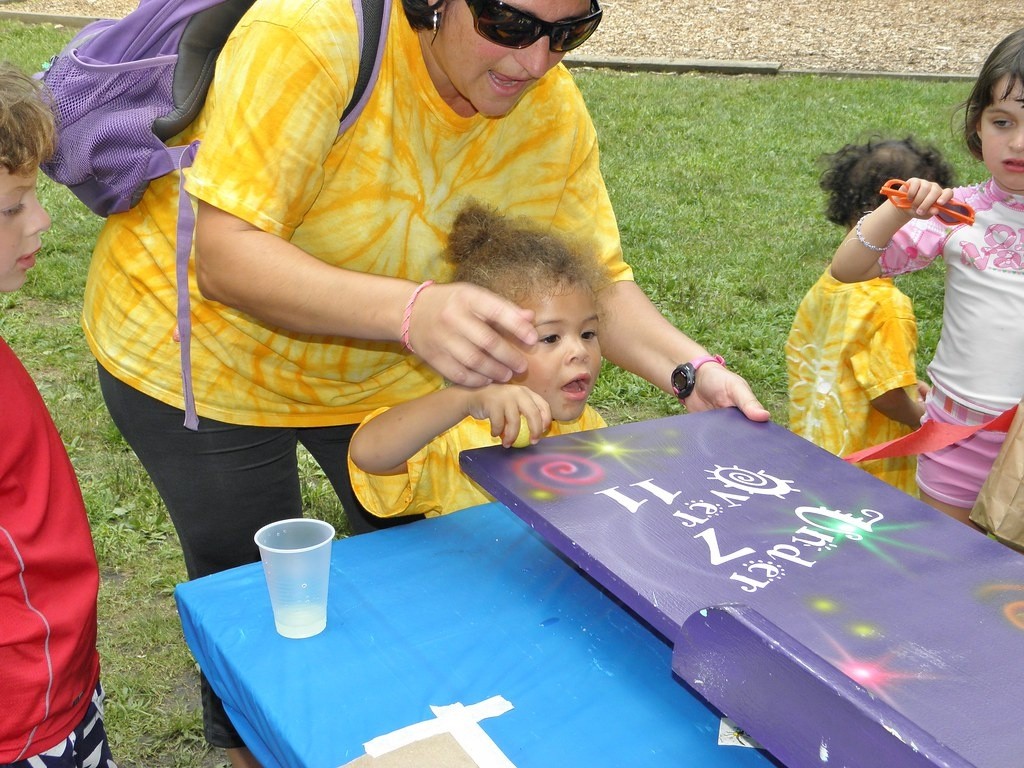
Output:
[[671, 355, 725, 406]]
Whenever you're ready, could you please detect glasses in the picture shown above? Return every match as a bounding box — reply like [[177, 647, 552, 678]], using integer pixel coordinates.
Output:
[[463, 1, 604, 53], [879, 179, 976, 227]]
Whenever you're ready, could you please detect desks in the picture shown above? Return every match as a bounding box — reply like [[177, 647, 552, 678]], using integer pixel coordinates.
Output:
[[172, 497, 783, 768]]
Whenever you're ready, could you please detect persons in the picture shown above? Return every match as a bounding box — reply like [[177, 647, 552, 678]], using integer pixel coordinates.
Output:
[[347, 201, 608, 519], [785, 134, 957, 501], [82, 1, 769, 768], [831, 27, 1024, 554], [0, 61, 118, 768]]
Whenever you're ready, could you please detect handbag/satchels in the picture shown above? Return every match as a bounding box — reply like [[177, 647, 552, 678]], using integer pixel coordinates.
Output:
[[844, 393, 1024, 554]]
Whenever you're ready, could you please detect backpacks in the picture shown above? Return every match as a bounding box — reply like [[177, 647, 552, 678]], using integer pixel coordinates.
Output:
[[27, 0, 395, 428]]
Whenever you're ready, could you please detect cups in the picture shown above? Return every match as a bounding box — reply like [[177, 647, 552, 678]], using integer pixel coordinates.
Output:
[[254, 518, 336, 638]]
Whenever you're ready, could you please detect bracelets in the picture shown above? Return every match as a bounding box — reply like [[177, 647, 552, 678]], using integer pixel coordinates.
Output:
[[856, 213, 894, 251], [402, 279, 434, 354]]
